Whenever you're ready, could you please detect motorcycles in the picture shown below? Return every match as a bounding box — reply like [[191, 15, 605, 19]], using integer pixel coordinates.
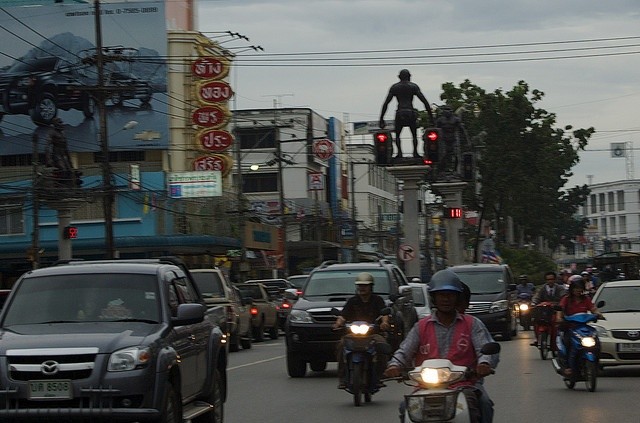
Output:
[[374, 341, 501, 422], [517, 296, 531, 330], [535, 298, 558, 360], [331, 307, 391, 406], [554, 300, 606, 392]]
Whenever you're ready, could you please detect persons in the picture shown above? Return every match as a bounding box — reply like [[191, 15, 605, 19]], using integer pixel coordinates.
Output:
[[334, 273, 391, 386], [532, 273, 560, 345], [378, 68, 435, 156], [555, 260, 631, 287], [388, 269, 489, 422], [558, 274, 603, 376], [458, 281, 499, 423], [513, 274, 536, 317]]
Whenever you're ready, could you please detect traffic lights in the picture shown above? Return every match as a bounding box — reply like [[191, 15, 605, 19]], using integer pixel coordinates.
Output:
[[374, 131, 392, 167], [423, 127, 439, 164], [444, 206, 461, 218], [64, 226, 77, 240]]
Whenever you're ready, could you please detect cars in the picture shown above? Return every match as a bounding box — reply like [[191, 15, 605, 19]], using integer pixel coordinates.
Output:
[[235, 283, 278, 341], [189, 268, 252, 352], [591, 279, 640, 374], [245, 278, 301, 327], [407, 283, 432, 318]]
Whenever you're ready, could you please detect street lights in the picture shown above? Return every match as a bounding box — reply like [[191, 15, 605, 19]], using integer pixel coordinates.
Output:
[[238, 165, 258, 239], [100, 121, 137, 259]]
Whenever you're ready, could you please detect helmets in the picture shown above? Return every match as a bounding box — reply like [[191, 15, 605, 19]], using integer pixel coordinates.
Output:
[[567, 275, 585, 288], [518, 275, 527, 279], [355, 273, 374, 285], [428, 270, 464, 293]]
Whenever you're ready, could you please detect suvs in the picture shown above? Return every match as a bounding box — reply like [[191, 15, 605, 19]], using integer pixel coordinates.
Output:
[[285, 259, 418, 377], [0, 256, 226, 423], [448, 264, 517, 340]]
